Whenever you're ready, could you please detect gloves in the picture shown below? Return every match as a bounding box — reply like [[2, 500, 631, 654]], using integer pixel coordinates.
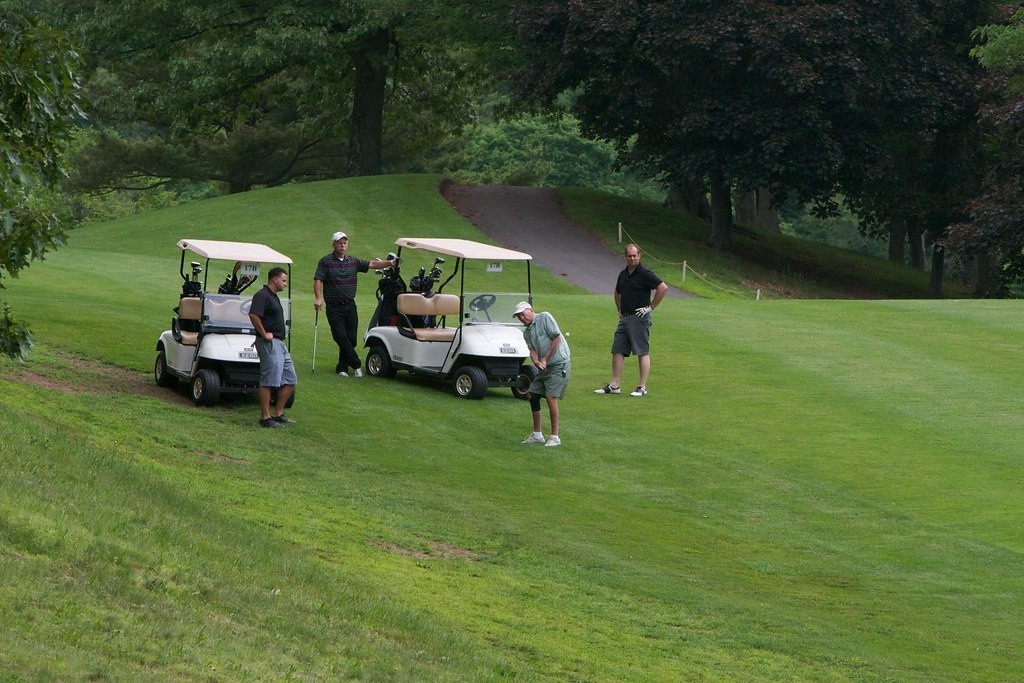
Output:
[[390, 257, 403, 266], [635, 305, 653, 319]]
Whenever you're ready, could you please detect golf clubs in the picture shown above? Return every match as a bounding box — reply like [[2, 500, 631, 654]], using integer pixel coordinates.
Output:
[[433, 257, 445, 268], [312, 307, 319, 373], [544, 369, 567, 378], [419, 267, 425, 280], [375, 270, 383, 278]]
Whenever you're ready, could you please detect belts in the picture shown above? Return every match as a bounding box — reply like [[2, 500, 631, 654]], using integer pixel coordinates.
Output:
[[622, 311, 632, 316]]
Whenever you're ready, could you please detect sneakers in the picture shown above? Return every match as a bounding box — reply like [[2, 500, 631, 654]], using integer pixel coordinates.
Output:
[[260, 415, 282, 429], [521, 433, 545, 443], [630, 383, 647, 396], [594, 381, 620, 394], [544, 434, 561, 447], [275, 413, 296, 423]]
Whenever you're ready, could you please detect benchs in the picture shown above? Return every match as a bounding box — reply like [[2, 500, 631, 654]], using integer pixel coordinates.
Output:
[[172, 297, 251, 345], [397, 293, 460, 342]]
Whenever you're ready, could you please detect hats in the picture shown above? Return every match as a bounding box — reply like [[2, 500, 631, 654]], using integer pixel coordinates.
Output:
[[512, 301, 532, 319], [332, 231, 349, 242]]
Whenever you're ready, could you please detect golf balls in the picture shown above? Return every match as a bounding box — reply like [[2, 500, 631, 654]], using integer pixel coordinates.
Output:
[[565, 332, 570, 336]]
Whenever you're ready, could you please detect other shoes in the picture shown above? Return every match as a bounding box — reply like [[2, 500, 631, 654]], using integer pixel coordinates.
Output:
[[354, 367, 362, 378], [339, 371, 349, 377]]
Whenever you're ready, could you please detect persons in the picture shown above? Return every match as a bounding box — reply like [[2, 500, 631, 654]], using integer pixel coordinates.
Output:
[[313, 231, 403, 379], [593, 243, 668, 396], [248, 267, 298, 427], [512, 301, 571, 447]]
